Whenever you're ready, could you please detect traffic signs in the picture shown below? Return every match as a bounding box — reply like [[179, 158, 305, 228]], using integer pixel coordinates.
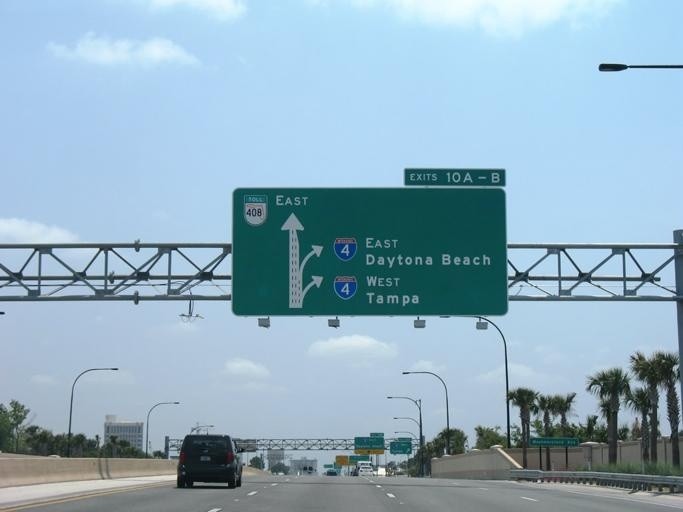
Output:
[[404, 166, 504, 187], [389, 441, 411, 454], [354, 437, 384, 454], [369, 433, 384, 437], [398, 438, 412, 441], [528, 436, 579, 448], [230, 185, 508, 317], [324, 456, 369, 468]]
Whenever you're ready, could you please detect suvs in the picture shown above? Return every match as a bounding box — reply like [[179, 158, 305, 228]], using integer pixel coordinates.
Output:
[[175, 433, 243, 489]]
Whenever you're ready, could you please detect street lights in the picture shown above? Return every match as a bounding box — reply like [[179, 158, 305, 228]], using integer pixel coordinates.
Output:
[[386, 396, 423, 477], [145, 400, 179, 457], [66, 367, 118, 457], [598, 63, 682, 75], [440, 314, 511, 448], [402, 370, 451, 455], [190, 424, 213, 435]]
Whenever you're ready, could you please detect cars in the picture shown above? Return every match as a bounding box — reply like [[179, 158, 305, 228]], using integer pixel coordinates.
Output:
[[325, 460, 373, 477]]
